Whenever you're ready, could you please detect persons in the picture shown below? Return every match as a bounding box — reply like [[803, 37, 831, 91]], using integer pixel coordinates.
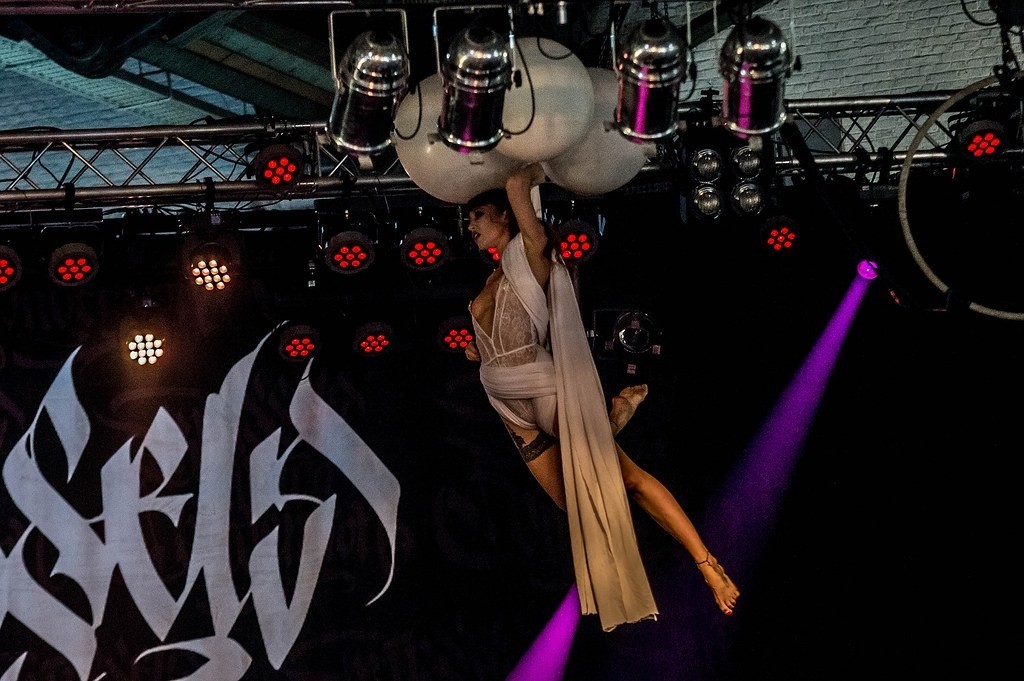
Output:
[[465, 163, 740, 633]]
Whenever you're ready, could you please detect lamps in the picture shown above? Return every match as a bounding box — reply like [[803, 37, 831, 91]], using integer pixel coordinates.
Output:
[[253, 130, 307, 196], [759, 206, 798, 252], [0, 216, 606, 381], [325, 30, 411, 154], [717, 17, 793, 138], [612, 20, 688, 145], [956, 107, 1005, 160], [436, 27, 518, 153]]
